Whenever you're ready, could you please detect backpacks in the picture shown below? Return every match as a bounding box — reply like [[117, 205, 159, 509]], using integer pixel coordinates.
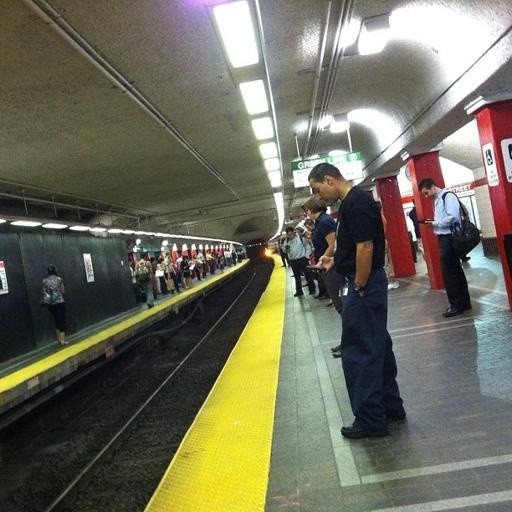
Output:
[[137, 263, 151, 282]]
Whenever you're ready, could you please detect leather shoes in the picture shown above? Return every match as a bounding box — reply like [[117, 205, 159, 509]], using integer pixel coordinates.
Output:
[[443, 307, 465, 317]]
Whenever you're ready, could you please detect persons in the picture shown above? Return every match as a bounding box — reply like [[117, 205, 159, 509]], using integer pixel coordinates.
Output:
[[126, 250, 245, 308], [300, 195, 344, 357], [43, 265, 69, 345], [375, 198, 401, 291], [277, 219, 330, 300], [308, 163, 408, 439], [461, 256, 472, 262], [405, 197, 433, 277], [419, 179, 474, 318]]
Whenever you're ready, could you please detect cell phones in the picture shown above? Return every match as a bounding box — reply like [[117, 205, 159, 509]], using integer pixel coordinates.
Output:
[[306, 265, 326, 272]]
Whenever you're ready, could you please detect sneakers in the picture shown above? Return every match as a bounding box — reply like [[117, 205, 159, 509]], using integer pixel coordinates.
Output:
[[342, 420, 389, 437], [387, 411, 406, 421], [463, 257, 470, 262], [331, 345, 342, 356], [294, 290, 304, 296], [309, 288, 333, 307], [387, 282, 400, 289]]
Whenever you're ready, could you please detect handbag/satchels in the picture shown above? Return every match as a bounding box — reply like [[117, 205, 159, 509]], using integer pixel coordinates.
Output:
[[155, 270, 164, 278], [451, 221, 480, 258], [39, 289, 51, 305], [165, 279, 175, 291]]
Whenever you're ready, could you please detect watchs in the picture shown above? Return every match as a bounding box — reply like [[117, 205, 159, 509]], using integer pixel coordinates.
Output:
[[353, 282, 367, 292]]
